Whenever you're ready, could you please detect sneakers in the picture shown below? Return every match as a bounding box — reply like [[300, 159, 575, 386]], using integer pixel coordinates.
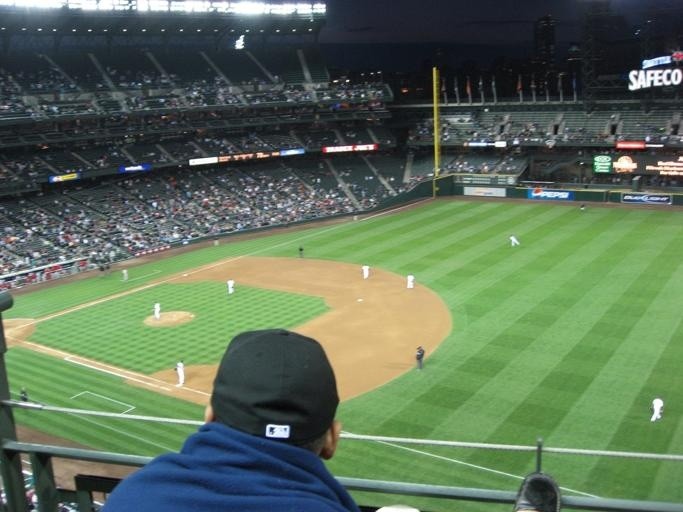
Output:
[[512, 472, 562, 512]]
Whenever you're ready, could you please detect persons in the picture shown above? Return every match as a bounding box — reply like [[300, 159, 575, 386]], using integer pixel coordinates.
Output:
[[416, 346, 426, 371], [20, 386, 26, 400], [651, 396, 665, 423], [1, 65, 682, 319], [93, 330, 562, 512], [176, 359, 184, 387]]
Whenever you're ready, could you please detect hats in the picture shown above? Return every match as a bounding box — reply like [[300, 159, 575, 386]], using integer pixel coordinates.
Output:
[[211, 327, 341, 447]]
